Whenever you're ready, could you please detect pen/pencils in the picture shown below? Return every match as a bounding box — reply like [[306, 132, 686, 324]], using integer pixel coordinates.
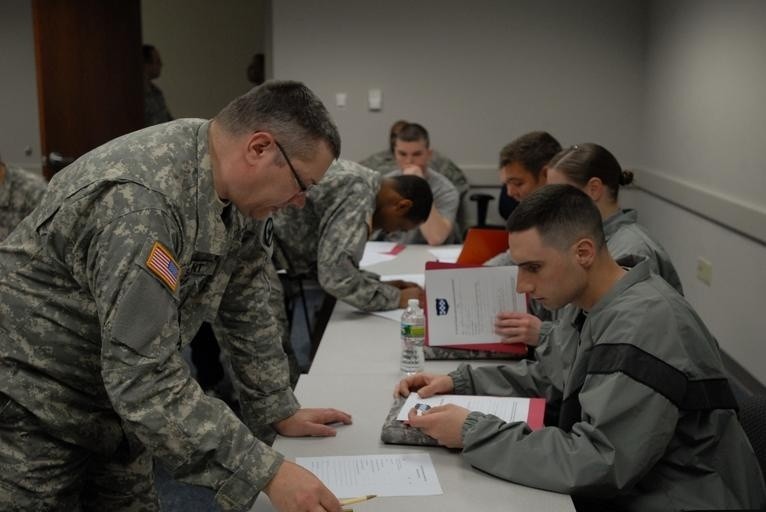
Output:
[[340, 494, 377, 505]]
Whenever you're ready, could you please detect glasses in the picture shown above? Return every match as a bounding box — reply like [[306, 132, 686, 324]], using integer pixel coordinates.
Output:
[[274, 138, 318, 198]]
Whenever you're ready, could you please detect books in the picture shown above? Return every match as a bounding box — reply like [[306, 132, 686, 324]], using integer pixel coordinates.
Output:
[[418, 262, 531, 361], [382, 387, 547, 449]]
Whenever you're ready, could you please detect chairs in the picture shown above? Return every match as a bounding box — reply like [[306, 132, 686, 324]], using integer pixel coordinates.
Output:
[[716, 348, 766, 485]]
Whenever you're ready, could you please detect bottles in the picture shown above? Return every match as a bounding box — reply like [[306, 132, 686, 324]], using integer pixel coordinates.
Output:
[[398, 297, 426, 376]]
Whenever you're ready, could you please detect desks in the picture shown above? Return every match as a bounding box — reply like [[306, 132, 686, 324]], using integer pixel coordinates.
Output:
[[247, 374, 578, 511], [307, 242, 526, 374]]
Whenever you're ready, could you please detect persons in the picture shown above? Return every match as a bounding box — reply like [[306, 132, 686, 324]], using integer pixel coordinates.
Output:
[[144, 43, 173, 126], [484, 140, 685, 360], [269, 160, 434, 390], [0, 79, 353, 512], [361, 118, 561, 250], [246, 53, 265, 87], [394, 184, 764, 510], [2, 162, 45, 242]]
